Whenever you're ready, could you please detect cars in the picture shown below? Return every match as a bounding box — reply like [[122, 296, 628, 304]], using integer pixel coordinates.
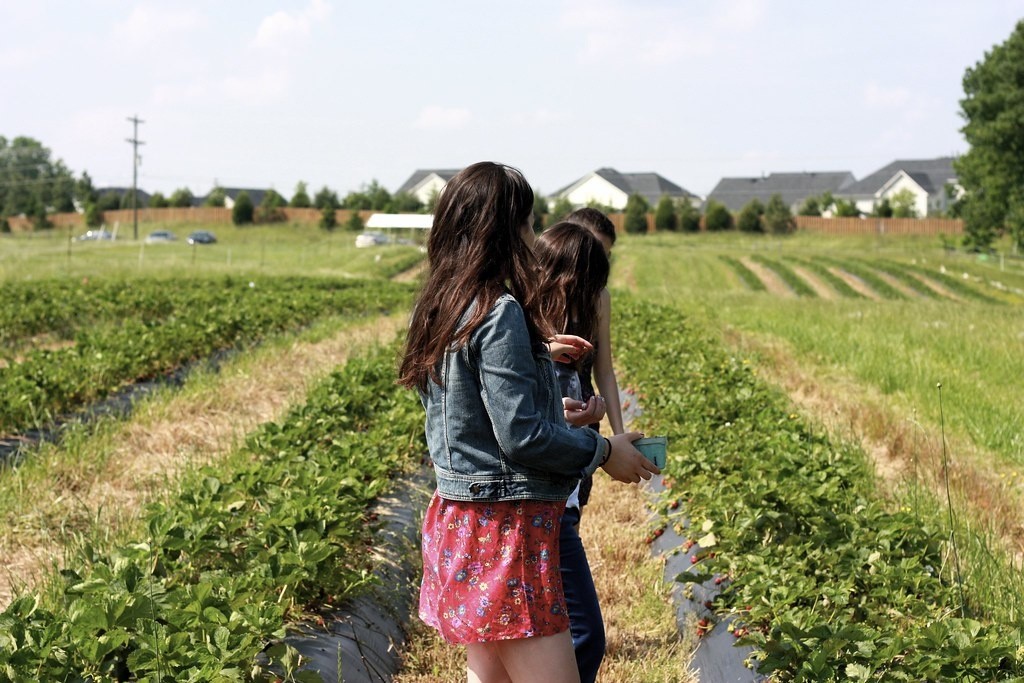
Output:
[[355, 231, 388, 247], [70, 231, 112, 240], [186, 232, 218, 244], [145, 232, 173, 242]]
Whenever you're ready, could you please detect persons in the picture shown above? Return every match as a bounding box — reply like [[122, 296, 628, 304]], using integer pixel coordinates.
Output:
[[396, 162, 661, 683]]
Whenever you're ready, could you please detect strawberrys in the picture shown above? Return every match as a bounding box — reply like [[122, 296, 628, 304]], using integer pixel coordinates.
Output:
[[621, 389, 755, 637]]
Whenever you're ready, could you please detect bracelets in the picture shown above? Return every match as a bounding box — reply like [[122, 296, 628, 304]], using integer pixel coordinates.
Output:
[[598, 437, 612, 468]]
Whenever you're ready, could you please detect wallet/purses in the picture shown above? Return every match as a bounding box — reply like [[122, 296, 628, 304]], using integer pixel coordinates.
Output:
[[632, 436, 667, 469]]
